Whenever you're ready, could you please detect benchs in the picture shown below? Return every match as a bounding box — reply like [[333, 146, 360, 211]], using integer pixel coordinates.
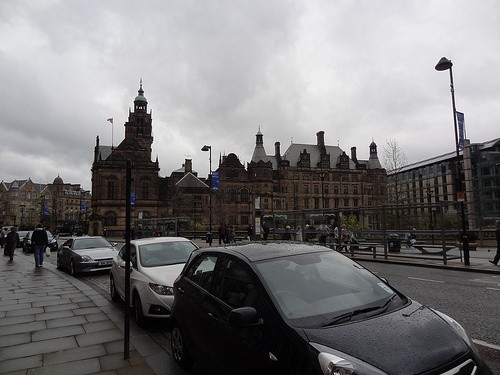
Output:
[[413, 244, 455, 254], [354, 242, 378, 250]]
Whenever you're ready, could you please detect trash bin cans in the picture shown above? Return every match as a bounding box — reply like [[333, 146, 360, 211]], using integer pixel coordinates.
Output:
[[206, 233, 213, 243], [388, 233, 401, 253], [3, 237, 15, 255]]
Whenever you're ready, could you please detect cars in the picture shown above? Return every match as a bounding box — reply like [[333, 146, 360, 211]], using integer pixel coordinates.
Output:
[[110, 236, 215, 326], [23, 229, 58, 253], [6, 231, 28, 247], [169, 239, 492, 375], [57, 235, 120, 277]]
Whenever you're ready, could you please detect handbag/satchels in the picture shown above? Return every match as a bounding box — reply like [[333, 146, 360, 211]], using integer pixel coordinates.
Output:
[[46, 246, 51, 257]]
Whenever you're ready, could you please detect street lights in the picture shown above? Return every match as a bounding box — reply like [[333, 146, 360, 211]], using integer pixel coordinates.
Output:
[[426, 190, 435, 245], [435, 54, 470, 265], [201, 144, 213, 246], [119, 145, 137, 360], [317, 171, 327, 217], [19, 204, 26, 231]]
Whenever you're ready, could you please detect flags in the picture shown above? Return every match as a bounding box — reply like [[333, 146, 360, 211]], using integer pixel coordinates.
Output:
[[108, 119, 113, 123]]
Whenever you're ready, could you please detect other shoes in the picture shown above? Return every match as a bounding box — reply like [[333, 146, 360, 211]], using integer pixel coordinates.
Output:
[[36, 265, 39, 267], [40, 263, 43, 265], [489, 260, 497, 265], [8, 259, 13, 262]]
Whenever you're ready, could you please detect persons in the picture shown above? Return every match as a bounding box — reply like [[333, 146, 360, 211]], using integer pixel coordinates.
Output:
[[0, 227, 5, 248], [248, 224, 253, 240], [218, 223, 236, 245], [31, 224, 48, 267], [489, 220, 500, 265], [262, 222, 269, 240], [407, 227, 417, 248], [306, 221, 358, 252], [5, 227, 19, 262], [275, 225, 302, 241]]
